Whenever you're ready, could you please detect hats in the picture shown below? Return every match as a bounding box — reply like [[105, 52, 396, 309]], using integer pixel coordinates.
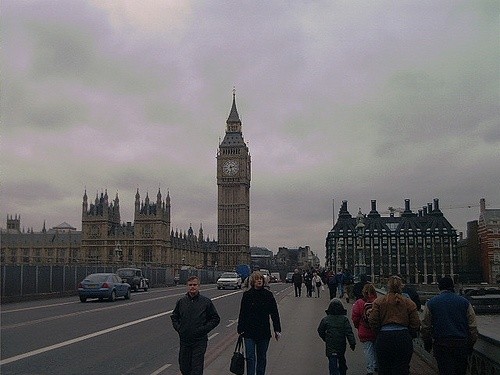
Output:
[[438, 277, 454, 290]]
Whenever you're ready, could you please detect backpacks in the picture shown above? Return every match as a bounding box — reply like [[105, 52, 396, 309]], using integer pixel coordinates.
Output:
[[362, 299, 372, 327]]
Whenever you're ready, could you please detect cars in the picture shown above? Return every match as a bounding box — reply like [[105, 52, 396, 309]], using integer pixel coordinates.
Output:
[[78, 273, 132, 302], [216, 272, 242, 290], [286, 273, 294, 283]]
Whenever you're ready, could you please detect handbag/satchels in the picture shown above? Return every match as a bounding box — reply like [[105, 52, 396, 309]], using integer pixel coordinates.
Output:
[[230, 336, 245, 375], [316, 282, 321, 287]]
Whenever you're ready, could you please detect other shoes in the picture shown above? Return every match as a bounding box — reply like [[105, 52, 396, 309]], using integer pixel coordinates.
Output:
[[373, 364, 379, 371], [366, 369, 374, 375]]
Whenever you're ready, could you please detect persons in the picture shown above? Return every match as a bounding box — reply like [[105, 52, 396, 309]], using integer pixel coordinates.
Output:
[[350, 273, 368, 299], [237, 271, 282, 375], [369, 275, 420, 375], [292, 269, 302, 297], [400, 279, 420, 311], [352, 284, 378, 375], [421, 277, 478, 375], [170, 276, 221, 375], [317, 299, 356, 375], [304, 266, 354, 303]]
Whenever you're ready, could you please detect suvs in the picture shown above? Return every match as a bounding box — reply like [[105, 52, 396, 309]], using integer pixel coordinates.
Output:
[[117, 268, 150, 292]]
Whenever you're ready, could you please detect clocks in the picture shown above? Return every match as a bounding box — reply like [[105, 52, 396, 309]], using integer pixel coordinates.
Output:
[[222, 160, 240, 176]]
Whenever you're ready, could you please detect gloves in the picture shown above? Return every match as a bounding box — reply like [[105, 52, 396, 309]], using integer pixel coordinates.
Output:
[[424, 338, 433, 353]]
